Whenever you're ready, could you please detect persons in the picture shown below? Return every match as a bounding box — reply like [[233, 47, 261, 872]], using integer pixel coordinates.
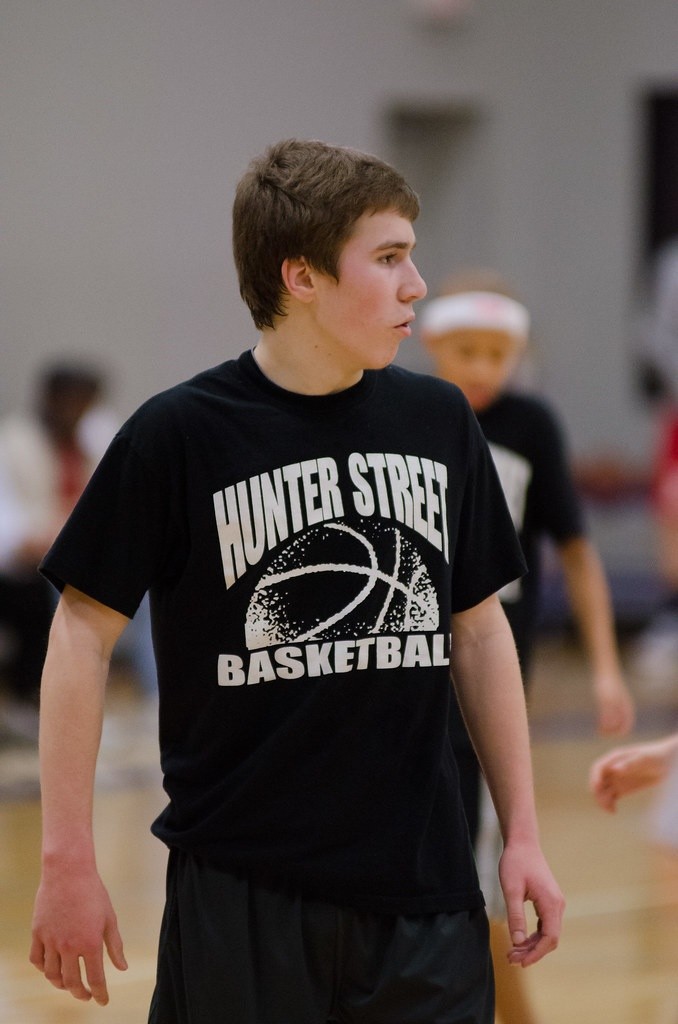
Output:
[[591, 732, 678, 813], [415, 271, 633, 1024], [0, 365, 104, 795], [24, 138, 566, 1024], [623, 329, 678, 692]]
